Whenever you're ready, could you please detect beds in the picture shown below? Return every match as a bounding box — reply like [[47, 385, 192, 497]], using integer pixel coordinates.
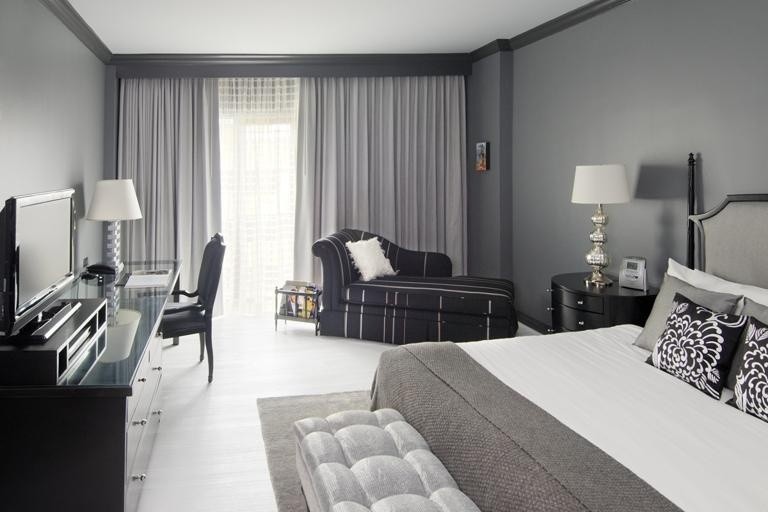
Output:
[[369, 152, 768, 512]]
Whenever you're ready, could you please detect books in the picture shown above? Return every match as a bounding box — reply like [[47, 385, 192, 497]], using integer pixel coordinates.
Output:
[[125, 267, 172, 289]]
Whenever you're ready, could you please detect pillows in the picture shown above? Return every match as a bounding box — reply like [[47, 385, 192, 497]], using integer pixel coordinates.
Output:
[[724, 296, 768, 391], [345, 236, 399, 282], [632, 272, 744, 353], [724, 316, 768, 422], [644, 292, 749, 402], [667, 257, 767, 308]]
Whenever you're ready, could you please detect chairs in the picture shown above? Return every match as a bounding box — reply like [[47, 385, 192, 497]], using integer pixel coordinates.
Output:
[[159, 232, 227, 383], [311, 228, 518, 345]]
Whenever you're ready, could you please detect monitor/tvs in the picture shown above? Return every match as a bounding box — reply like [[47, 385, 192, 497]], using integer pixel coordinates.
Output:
[[0, 188, 82, 344]]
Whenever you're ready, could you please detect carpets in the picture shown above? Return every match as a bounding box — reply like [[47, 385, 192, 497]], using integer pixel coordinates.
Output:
[[256, 390, 373, 512]]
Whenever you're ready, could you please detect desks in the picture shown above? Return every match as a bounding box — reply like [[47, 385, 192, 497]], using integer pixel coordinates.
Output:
[[0, 260, 185, 512]]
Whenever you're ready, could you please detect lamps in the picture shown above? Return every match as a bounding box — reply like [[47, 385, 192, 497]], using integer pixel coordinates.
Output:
[[570, 163, 631, 288], [85, 179, 144, 276]]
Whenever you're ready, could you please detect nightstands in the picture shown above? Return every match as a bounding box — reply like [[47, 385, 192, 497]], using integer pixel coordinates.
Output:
[[545, 271, 661, 334]]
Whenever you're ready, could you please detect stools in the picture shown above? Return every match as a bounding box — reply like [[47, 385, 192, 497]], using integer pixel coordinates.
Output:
[[293, 407, 482, 512]]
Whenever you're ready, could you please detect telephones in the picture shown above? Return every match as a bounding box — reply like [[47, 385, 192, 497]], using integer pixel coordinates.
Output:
[[81, 263, 118, 282]]
